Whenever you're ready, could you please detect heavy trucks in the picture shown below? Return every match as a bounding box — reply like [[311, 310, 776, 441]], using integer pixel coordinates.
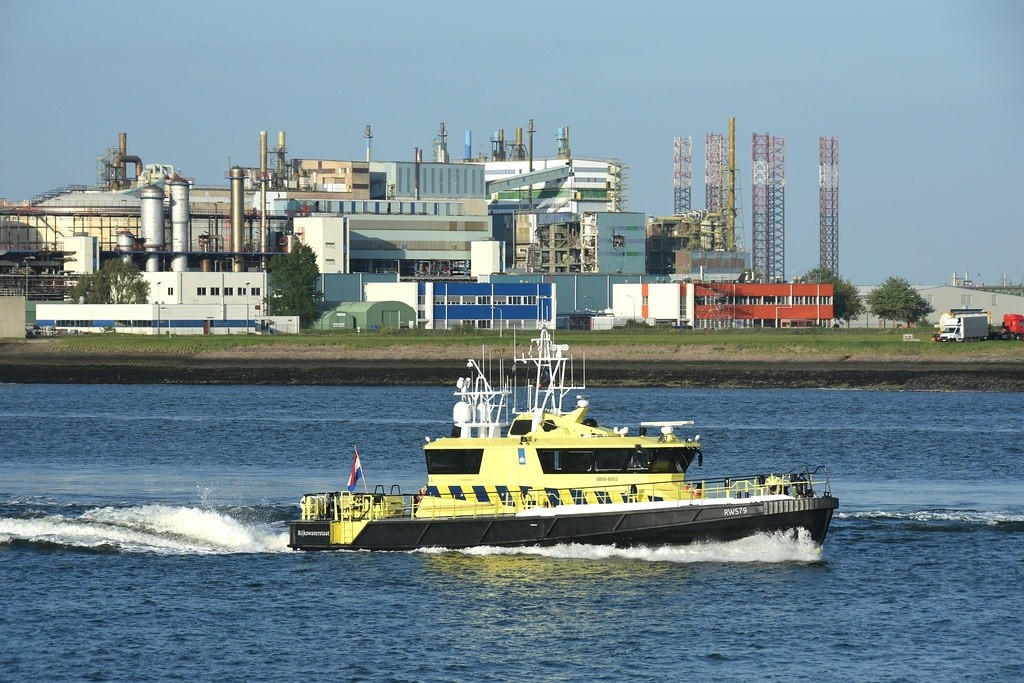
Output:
[[987, 313, 1024, 341]]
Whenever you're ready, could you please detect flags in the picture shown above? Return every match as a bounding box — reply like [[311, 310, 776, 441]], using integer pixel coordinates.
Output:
[[347, 454, 362, 492]]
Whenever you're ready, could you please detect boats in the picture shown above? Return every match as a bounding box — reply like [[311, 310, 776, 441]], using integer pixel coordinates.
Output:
[[283, 321, 839, 550]]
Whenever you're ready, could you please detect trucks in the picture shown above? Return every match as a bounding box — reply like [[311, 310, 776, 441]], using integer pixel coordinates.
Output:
[[940, 313, 988, 342]]
